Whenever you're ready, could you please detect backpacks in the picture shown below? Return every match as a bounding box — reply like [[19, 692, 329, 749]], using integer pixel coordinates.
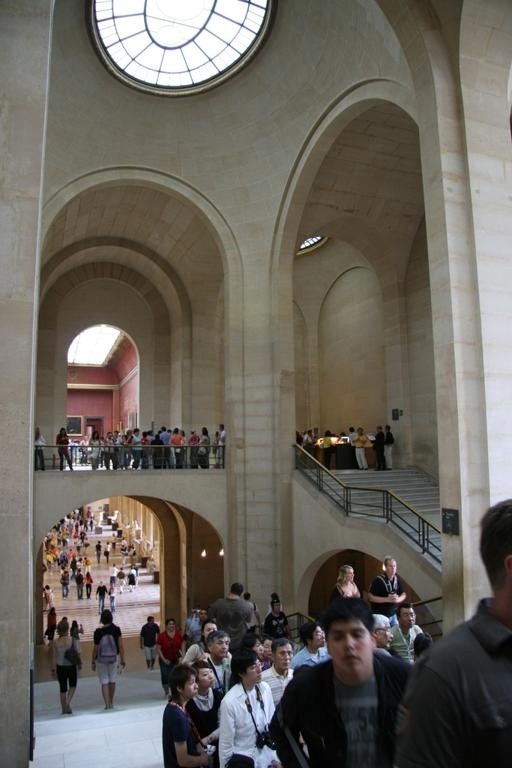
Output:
[[98, 628, 118, 664]]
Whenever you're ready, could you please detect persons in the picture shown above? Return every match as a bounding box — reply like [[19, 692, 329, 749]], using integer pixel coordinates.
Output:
[[50, 621, 82, 715], [267, 556, 433, 767], [70, 620, 84, 654], [157, 582, 296, 768], [392, 497, 512, 768], [62, 617, 68, 636], [140, 615, 160, 669], [55, 427, 74, 471], [35, 424, 47, 471], [90, 609, 126, 710], [43, 506, 139, 613], [79, 424, 226, 472], [45, 607, 57, 641], [296, 424, 395, 470]]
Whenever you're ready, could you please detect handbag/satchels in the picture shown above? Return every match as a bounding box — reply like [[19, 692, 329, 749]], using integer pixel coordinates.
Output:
[[64, 648, 82, 665]]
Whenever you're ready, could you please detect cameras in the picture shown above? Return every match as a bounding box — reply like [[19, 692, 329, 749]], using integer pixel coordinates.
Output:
[[257, 732, 276, 749]]
